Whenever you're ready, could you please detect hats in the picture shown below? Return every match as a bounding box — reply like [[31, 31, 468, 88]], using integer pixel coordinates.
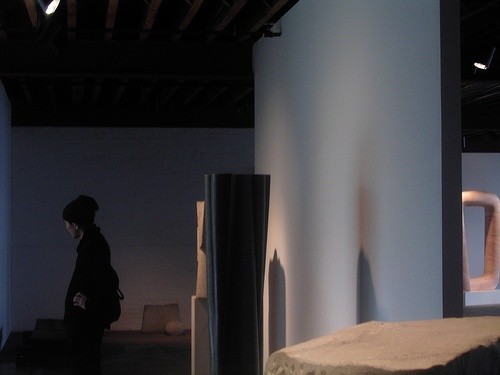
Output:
[[63, 194, 99, 228]]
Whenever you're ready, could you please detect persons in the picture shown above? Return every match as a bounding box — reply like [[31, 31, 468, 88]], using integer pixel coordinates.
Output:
[[60, 196, 121, 375]]
